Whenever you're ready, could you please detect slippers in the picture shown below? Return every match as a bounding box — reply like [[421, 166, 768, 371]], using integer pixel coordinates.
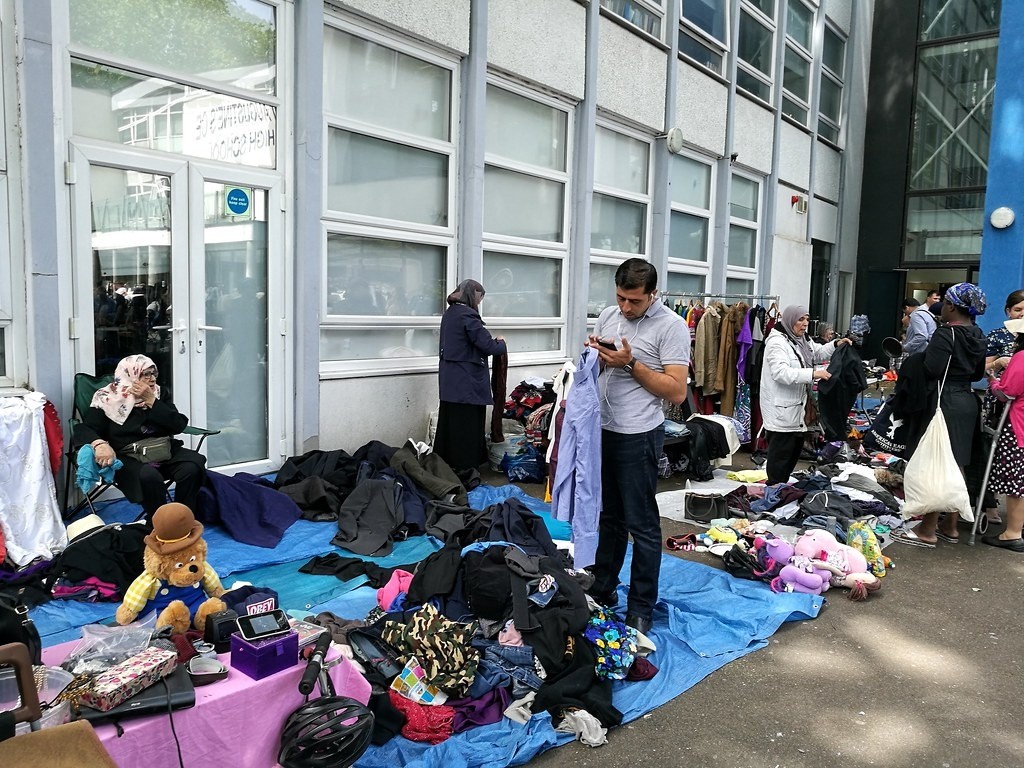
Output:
[[935, 525, 959, 544], [889, 527, 937, 549]]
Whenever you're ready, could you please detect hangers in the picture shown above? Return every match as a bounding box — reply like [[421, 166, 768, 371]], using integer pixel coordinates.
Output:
[[666, 290, 782, 319]]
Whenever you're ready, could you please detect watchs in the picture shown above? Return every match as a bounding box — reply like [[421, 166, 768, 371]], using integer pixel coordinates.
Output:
[[622, 357, 637, 374]]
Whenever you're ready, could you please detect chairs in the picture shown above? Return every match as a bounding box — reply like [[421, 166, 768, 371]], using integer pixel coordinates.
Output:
[[62, 372, 220, 523]]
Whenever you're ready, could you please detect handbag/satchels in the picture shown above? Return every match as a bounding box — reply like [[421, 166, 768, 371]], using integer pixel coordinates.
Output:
[[723, 543, 763, 580], [123, 437, 173, 464], [501, 445, 546, 484], [1, 592, 44, 666], [902, 406, 975, 524], [847, 522, 886, 578], [684, 491, 730, 522], [864, 400, 908, 462]]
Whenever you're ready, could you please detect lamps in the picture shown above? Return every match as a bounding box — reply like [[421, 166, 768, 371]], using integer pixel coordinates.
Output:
[[666, 127, 683, 154], [990, 207, 1014, 228]]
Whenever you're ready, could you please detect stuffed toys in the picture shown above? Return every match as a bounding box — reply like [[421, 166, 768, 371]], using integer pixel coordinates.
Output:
[[695, 517, 882, 601], [114, 503, 228, 633]]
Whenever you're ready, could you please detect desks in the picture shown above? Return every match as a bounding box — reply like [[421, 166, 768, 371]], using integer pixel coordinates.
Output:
[[663, 418, 701, 482], [0, 623, 373, 768]]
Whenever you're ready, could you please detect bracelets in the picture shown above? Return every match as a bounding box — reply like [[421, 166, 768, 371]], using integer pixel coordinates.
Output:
[[93, 441, 109, 450]]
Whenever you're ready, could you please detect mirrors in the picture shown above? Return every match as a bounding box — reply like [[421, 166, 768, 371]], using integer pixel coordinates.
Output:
[[881, 336, 903, 369]]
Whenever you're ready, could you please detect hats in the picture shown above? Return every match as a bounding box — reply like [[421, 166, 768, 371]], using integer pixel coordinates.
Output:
[[61, 514, 124, 553]]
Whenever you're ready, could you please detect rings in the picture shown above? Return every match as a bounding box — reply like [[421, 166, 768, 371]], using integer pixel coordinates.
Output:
[[135, 389, 137, 391], [98, 462, 102, 464], [825, 377, 827, 378]]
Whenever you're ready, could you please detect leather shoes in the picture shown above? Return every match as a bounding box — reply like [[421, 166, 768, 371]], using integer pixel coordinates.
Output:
[[625, 614, 653, 635], [604, 590, 619, 608]]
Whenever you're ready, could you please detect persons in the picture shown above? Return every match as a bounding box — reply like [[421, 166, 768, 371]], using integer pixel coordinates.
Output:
[[888, 282, 989, 549], [814, 322, 835, 343], [433, 279, 507, 472], [205, 275, 269, 443], [93, 279, 171, 374], [980, 315, 1024, 552], [85, 354, 204, 526], [584, 259, 690, 634], [759, 304, 853, 486], [982, 288, 1024, 522]]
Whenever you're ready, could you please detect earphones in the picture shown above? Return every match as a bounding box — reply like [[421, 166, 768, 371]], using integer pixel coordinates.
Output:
[[650, 294, 654, 302]]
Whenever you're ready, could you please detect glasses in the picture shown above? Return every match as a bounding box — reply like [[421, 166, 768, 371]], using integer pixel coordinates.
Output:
[[144, 370, 158, 378]]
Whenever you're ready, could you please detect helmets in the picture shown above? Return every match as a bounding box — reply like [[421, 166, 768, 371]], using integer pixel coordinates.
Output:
[[277, 696, 373, 768]]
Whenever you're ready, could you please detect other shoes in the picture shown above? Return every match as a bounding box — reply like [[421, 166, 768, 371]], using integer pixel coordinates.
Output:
[[986, 510, 1003, 524], [981, 531, 1024, 552]]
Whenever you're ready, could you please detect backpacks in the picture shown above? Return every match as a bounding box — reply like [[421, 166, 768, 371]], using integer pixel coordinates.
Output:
[[918, 310, 946, 328]]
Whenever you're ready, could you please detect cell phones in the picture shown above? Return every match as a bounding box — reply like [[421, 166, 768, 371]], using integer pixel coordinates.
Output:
[[598, 340, 618, 352]]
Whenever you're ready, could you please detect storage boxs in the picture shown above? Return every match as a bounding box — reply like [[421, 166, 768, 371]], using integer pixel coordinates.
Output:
[[76, 646, 178, 712], [231, 630, 299, 682]]
